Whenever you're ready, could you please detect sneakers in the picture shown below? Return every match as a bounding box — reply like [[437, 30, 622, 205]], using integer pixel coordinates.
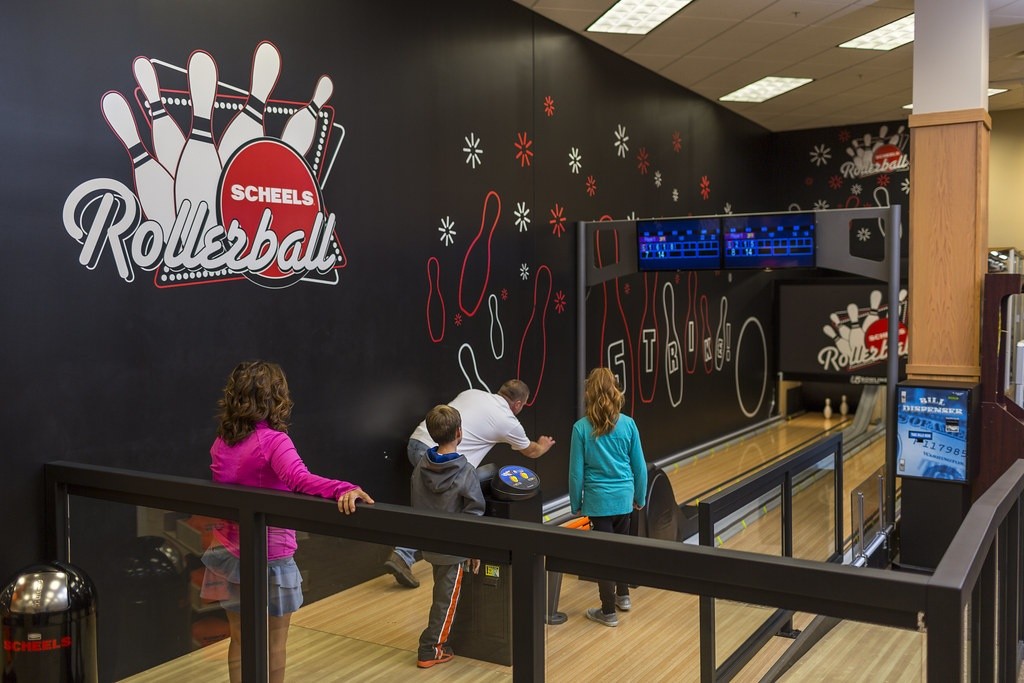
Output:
[[587, 607, 619, 626], [614, 593, 630, 610], [417, 646, 454, 668]]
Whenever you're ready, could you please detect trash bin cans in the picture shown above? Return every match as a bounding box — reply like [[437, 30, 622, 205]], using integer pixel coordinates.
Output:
[[0, 563, 102, 682]]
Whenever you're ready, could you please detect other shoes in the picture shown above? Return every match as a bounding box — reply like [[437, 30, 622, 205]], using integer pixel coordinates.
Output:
[[384, 552, 420, 588]]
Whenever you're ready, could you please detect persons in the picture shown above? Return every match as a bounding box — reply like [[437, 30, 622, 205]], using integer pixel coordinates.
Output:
[[409, 404, 486, 668], [384, 379, 556, 587], [569, 367, 649, 627], [199, 362, 374, 683]]
